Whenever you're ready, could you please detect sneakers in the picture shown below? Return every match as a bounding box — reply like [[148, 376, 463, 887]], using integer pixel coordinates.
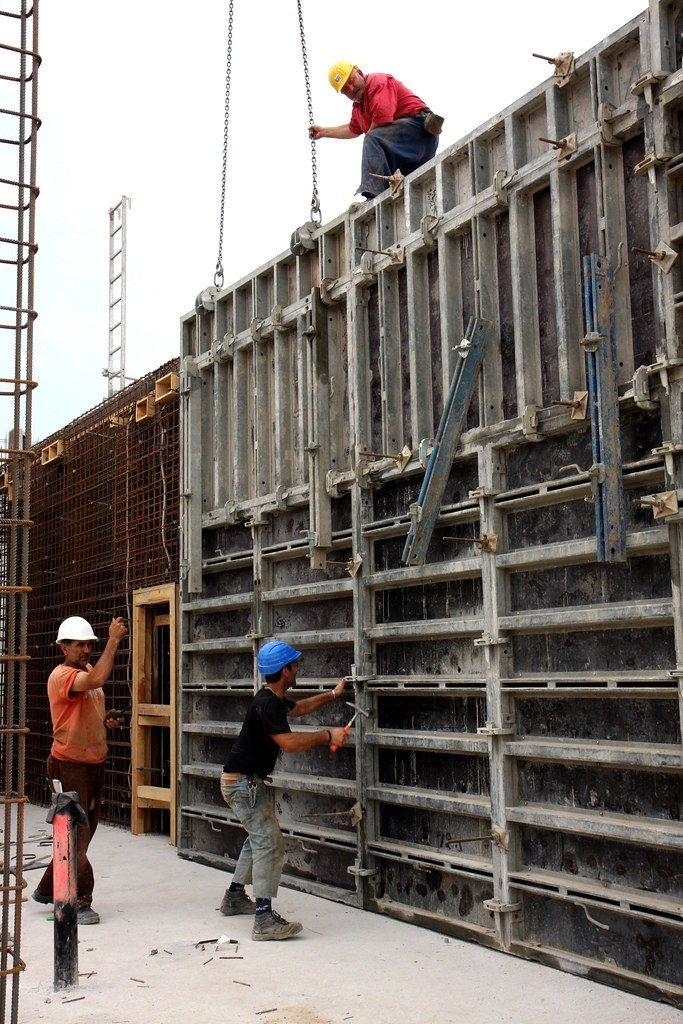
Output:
[[252, 910, 303, 941], [77, 904, 100, 924], [221, 889, 257, 915], [32, 889, 53, 904]]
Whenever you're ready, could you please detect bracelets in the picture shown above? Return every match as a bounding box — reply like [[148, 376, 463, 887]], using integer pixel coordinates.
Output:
[[327, 730, 332, 744], [332, 690, 335, 699]]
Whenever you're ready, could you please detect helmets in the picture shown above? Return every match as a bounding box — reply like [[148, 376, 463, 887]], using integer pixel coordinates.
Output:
[[256, 641, 301, 675], [329, 63, 353, 95], [56, 615, 99, 644]]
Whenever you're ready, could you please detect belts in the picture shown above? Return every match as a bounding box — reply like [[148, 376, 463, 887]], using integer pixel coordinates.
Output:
[[221, 772, 273, 783]]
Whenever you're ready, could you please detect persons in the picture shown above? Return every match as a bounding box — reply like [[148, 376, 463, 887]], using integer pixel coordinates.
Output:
[[30, 616, 127, 925], [308, 61, 439, 201], [219, 642, 353, 941]]
